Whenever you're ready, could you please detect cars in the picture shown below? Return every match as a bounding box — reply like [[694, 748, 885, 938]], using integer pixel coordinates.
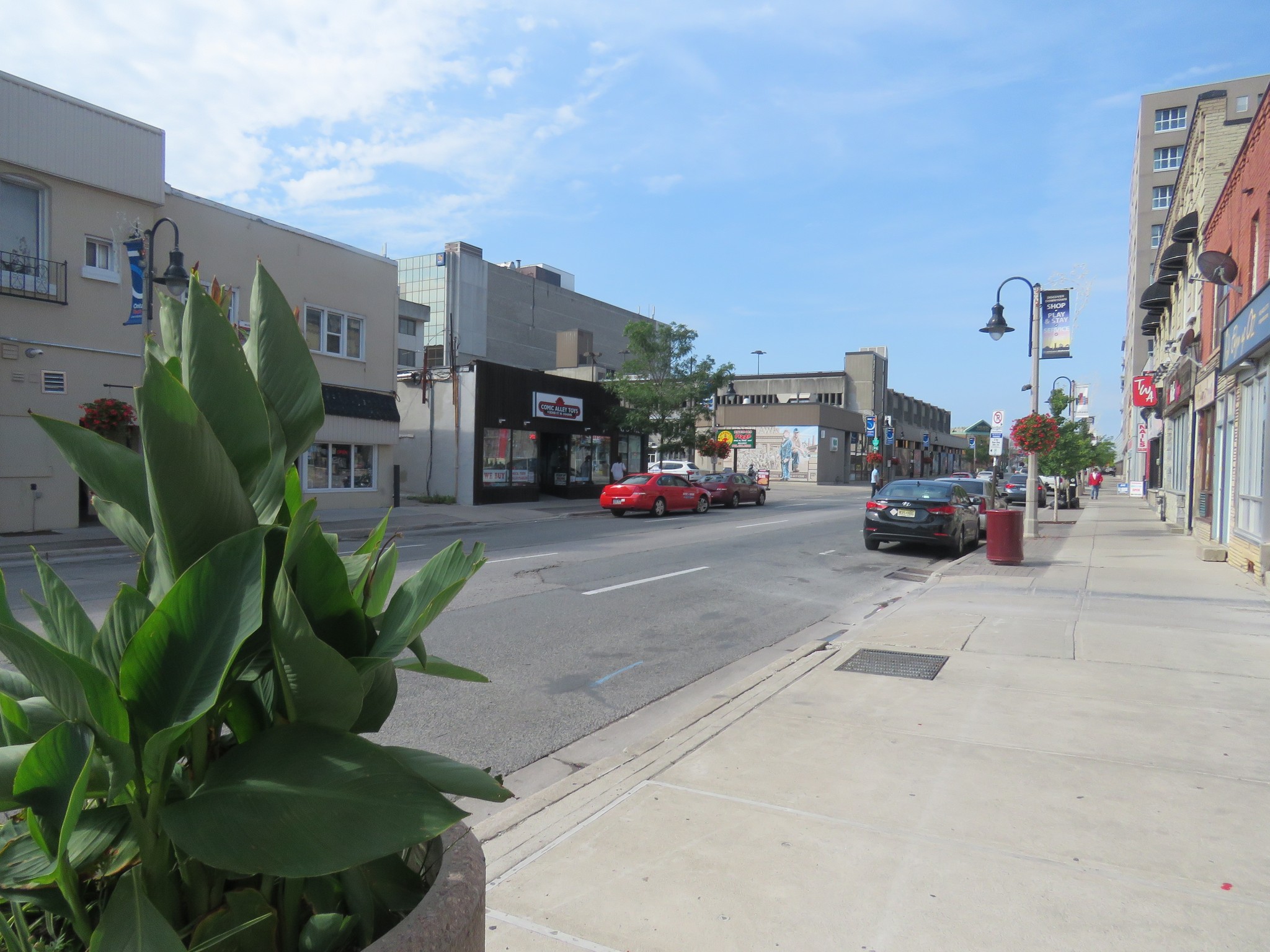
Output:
[[503, 457, 594, 485], [950, 464, 1089, 496], [647, 460, 703, 482], [1099, 467, 1114, 476], [600, 471, 711, 519], [864, 480, 983, 558], [932, 478, 1009, 531], [1004, 474, 1050, 507], [690, 472, 766, 508]]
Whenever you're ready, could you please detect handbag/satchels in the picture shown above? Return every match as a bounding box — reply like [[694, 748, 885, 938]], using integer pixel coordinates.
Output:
[[623, 470, 628, 477], [748, 473, 752, 476]]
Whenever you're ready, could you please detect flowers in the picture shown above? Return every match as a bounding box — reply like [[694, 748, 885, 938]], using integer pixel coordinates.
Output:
[[77, 396, 137, 434], [1010, 412, 1060, 457]]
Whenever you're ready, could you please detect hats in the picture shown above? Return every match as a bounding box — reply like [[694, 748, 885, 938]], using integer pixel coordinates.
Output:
[[1093, 468, 1096, 470]]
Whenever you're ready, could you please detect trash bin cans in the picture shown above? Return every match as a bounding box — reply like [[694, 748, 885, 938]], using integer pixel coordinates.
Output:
[[879, 478, 884, 488], [986, 509, 1024, 566], [1069, 478, 1077, 501]]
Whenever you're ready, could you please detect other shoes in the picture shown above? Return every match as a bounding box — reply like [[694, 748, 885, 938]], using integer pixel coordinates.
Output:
[[1091, 496, 1093, 499], [1096, 497, 1097, 499]]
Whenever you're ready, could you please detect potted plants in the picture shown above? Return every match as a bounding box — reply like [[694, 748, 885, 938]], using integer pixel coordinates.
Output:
[[0, 251, 512, 952]]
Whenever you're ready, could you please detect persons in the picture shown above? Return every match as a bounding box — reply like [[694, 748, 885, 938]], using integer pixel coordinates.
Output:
[[870, 464, 881, 499], [579, 455, 592, 478], [1112, 465, 1117, 478], [611, 456, 627, 483], [999, 468, 1004, 479], [1088, 468, 1103, 500], [901, 486, 916, 498], [746, 464, 757, 482]]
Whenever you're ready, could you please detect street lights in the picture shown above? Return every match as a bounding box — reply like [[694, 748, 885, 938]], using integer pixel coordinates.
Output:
[[712, 369, 737, 474], [618, 350, 631, 362], [147, 219, 190, 322], [892, 424, 905, 480], [1044, 376, 1076, 431], [877, 411, 891, 468], [583, 351, 603, 485], [979, 276, 1041, 538], [751, 350, 767, 376], [687, 357, 695, 462], [926, 430, 938, 481]]
